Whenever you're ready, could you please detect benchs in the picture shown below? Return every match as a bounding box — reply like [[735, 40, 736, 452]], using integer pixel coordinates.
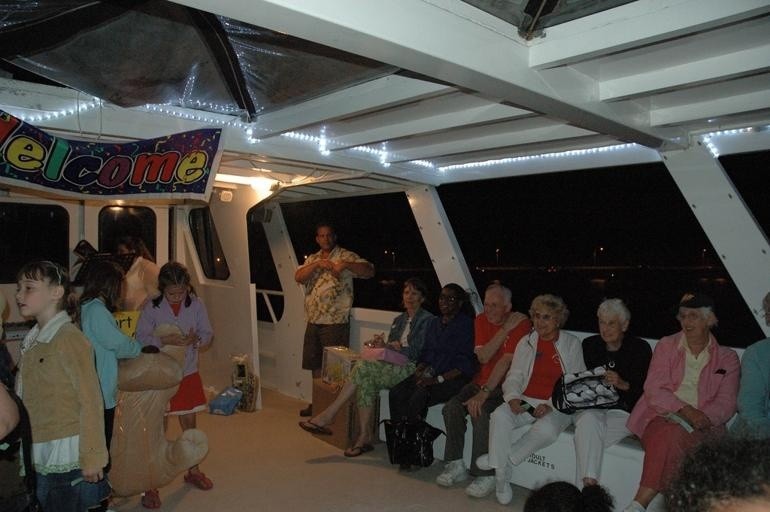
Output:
[[378, 389, 664, 512]]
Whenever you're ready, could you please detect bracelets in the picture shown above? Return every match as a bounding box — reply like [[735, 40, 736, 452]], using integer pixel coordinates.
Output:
[[481, 383, 493, 394], [679, 404, 692, 412], [347, 262, 353, 271]]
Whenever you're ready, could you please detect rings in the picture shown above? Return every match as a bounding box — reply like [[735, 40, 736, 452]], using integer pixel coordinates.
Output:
[[540, 410, 544, 415]]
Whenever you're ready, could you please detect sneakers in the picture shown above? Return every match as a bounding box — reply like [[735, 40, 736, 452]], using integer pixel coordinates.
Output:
[[299, 403, 312, 416], [142, 489, 161, 508], [436, 459, 467, 486], [184, 472, 213, 489], [466, 454, 513, 505]]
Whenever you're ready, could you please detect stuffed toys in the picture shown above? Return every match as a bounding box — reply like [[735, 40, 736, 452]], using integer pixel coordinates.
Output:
[[109, 323, 210, 508]]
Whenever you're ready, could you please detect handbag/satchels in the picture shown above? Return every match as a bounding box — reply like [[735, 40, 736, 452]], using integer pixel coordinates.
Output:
[[378, 419, 446, 467], [560, 364, 620, 409], [360, 347, 407, 366]]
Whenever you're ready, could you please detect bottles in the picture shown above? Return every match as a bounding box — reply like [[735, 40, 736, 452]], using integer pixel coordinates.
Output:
[[421, 365, 435, 379]]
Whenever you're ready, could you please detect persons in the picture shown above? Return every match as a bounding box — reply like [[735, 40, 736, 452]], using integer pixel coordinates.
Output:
[[134, 261, 215, 512], [387, 282, 481, 473], [294, 220, 374, 417], [298, 277, 435, 457], [78, 258, 142, 510], [523, 480, 616, 512], [434, 283, 534, 486], [14, 259, 109, 510], [476, 293, 587, 505], [621, 293, 742, 511], [570, 298, 654, 493], [64, 233, 162, 311], [729, 293, 769, 441], [661, 430, 770, 512], [0, 291, 36, 512]]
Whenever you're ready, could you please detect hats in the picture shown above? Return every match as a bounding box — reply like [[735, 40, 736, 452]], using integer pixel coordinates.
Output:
[[679, 293, 713, 309]]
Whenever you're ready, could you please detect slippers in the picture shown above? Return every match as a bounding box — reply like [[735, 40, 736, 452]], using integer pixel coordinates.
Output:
[[344, 445, 374, 457], [299, 421, 333, 435]]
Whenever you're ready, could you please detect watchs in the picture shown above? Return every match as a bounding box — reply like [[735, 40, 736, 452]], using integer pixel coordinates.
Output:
[[436, 375, 445, 383]]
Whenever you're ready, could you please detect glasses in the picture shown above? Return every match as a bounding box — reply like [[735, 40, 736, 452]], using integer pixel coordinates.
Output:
[[534, 312, 558, 321]]
